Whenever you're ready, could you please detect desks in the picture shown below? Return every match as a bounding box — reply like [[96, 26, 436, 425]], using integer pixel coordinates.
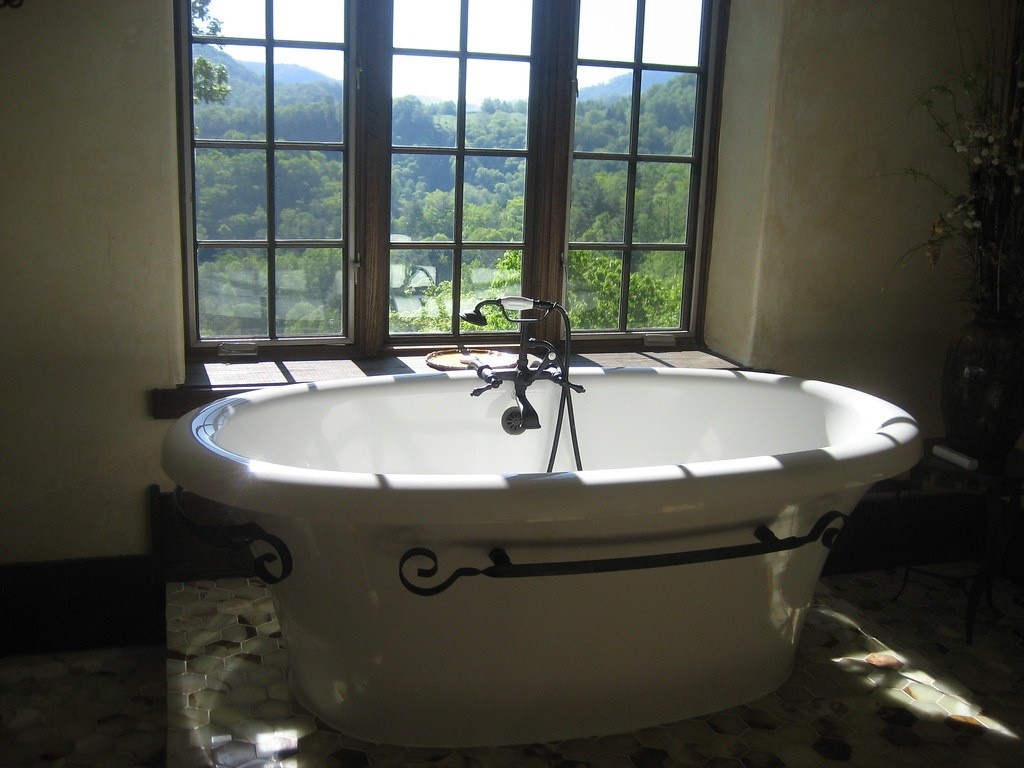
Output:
[[884, 439, 1024, 641]]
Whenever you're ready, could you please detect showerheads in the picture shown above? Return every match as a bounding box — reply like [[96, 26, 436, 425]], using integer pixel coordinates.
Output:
[[457, 295, 556, 328]]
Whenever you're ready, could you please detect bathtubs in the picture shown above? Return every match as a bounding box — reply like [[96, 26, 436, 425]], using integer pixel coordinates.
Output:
[[160, 362, 929, 752]]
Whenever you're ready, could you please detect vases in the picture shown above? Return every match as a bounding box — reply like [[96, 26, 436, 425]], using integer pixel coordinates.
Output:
[[939, 306, 1024, 475]]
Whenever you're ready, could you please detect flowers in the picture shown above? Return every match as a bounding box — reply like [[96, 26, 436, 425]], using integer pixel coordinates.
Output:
[[887, 0, 1024, 310]]
